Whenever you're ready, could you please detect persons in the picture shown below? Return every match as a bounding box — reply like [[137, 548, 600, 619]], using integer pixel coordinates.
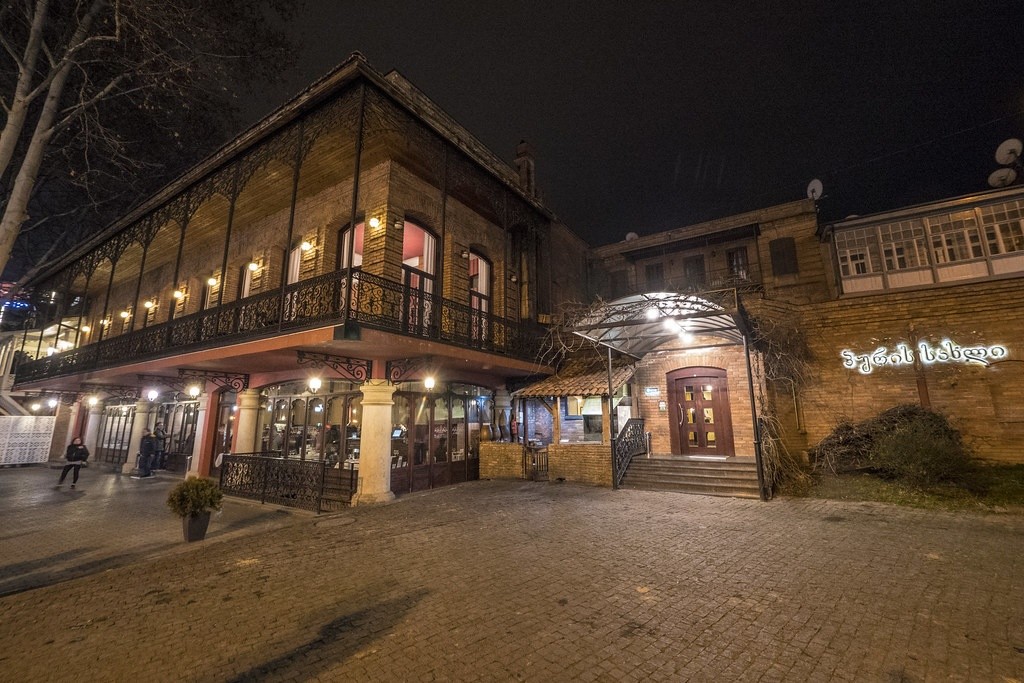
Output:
[[131, 429, 157, 479], [325, 423, 357, 451], [151, 421, 169, 472], [53, 437, 90, 490], [294, 431, 303, 454], [217, 424, 225, 454], [263, 424, 293, 449]]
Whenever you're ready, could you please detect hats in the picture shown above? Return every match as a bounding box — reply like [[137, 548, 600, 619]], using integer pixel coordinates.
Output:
[[219, 424, 226, 427]]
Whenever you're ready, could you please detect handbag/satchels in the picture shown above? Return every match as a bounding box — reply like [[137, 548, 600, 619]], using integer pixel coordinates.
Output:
[[81, 460, 89, 469], [130, 468, 144, 479]]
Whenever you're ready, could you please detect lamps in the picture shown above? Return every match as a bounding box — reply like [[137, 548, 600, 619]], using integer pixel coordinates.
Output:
[[511, 275, 517, 282], [395, 220, 404, 230], [461, 251, 468, 259]]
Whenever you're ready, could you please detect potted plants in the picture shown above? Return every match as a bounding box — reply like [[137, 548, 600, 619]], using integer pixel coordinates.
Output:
[[165, 475, 226, 543]]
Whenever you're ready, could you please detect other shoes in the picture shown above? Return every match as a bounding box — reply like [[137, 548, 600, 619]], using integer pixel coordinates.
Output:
[[69, 483, 76, 491], [141, 474, 155, 479], [155, 469, 166, 472], [53, 484, 63, 490]]
